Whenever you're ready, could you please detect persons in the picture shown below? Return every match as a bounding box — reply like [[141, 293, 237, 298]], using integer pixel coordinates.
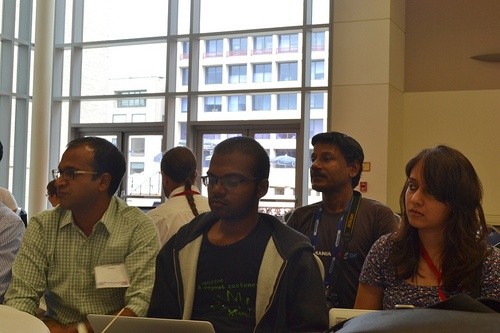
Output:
[[0, 137, 211, 333], [354, 145, 500, 309], [146, 136, 330, 333], [286, 131, 398, 309]]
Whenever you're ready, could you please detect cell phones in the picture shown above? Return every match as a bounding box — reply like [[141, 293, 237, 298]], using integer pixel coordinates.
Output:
[[394, 304, 416, 309]]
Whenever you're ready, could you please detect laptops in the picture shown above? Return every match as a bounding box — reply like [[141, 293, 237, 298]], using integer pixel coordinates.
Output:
[[328, 308, 376, 330], [87, 313, 216, 333]]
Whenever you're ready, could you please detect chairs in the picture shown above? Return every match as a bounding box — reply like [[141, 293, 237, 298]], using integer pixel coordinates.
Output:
[[0, 305, 50, 333], [329, 308, 383, 333]]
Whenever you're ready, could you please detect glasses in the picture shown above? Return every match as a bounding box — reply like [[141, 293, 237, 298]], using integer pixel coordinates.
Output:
[[201, 175, 262, 189], [52, 169, 100, 180], [46, 193, 50, 198]]
